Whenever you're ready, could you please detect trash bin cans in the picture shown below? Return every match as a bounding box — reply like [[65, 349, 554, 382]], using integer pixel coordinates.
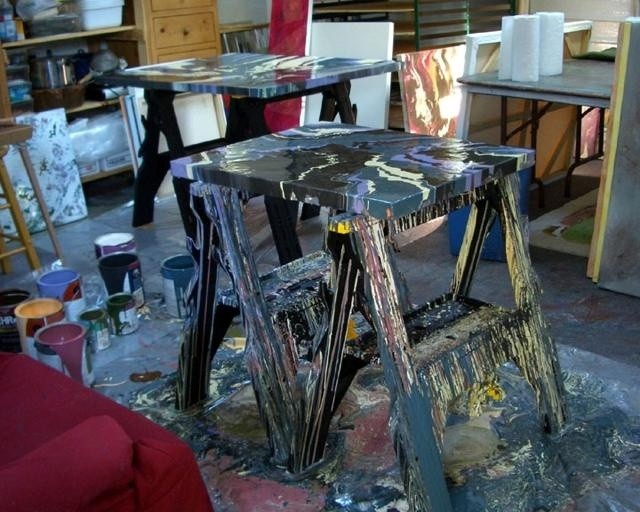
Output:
[[448, 169, 531, 262]]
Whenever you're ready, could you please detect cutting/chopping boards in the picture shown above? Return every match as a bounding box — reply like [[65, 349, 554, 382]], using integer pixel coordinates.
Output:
[[31, 86, 86, 113]]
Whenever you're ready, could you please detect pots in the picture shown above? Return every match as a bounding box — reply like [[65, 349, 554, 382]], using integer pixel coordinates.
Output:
[[32, 48, 76, 89]]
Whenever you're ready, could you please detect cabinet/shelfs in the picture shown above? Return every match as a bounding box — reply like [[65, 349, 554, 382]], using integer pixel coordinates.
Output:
[[3, 3, 142, 187], [105, 1, 221, 65]]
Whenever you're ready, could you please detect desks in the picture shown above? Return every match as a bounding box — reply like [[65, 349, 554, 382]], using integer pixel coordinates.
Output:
[[458, 60, 609, 207], [96, 55, 402, 239], [171, 122, 570, 512]]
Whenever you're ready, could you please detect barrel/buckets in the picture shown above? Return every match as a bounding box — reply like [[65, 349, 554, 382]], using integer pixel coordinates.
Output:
[[0, 233, 197, 388], [448, 143, 534, 260]]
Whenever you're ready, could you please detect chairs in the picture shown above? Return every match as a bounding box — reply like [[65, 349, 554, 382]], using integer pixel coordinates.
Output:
[[1, 351, 213, 506]]
